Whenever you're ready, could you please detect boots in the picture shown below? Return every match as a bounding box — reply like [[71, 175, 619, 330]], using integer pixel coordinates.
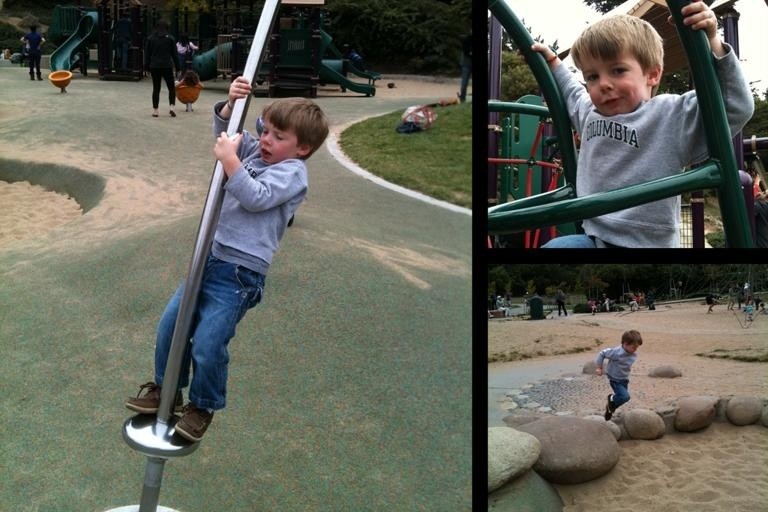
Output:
[[36, 72, 43, 80], [29, 72, 35, 80]]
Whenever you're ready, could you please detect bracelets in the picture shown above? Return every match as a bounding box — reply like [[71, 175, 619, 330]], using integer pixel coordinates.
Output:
[[545, 56, 557, 63], [227, 100, 232, 111], [597, 367, 602, 369]]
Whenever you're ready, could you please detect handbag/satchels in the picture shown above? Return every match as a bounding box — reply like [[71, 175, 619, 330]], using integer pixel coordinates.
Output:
[[396, 105, 437, 134], [184, 50, 193, 61]]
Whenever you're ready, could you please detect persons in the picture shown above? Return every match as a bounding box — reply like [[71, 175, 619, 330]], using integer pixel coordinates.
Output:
[[144, 20, 182, 117], [496, 296, 511, 318], [556, 288, 567, 316], [516, 0, 755, 249], [727, 281, 768, 326], [124, 75, 330, 443], [174, 70, 204, 111], [113, 14, 132, 73], [706, 294, 716, 313], [595, 330, 643, 421], [506, 289, 513, 307], [457, 34, 472, 104], [490, 290, 497, 310], [20, 23, 46, 81], [628, 290, 655, 311], [587, 293, 615, 315], [747, 167, 768, 199], [176, 32, 199, 79]]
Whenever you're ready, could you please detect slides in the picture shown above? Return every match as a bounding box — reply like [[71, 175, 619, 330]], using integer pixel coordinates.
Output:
[[320, 30, 382, 96], [48, 11, 98, 75], [190, 41, 233, 82]]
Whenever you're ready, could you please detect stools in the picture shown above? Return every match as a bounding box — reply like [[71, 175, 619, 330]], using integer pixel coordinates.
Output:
[[48, 70, 72, 93], [176, 85, 202, 112]]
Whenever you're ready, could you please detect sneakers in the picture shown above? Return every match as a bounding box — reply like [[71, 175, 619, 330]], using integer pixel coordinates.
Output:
[[175, 403, 213, 442], [605, 403, 612, 421], [608, 394, 616, 414], [125, 382, 183, 415], [152, 110, 176, 117]]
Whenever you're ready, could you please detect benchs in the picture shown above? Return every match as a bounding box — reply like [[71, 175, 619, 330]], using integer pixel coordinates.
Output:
[[490, 310, 505, 318]]
